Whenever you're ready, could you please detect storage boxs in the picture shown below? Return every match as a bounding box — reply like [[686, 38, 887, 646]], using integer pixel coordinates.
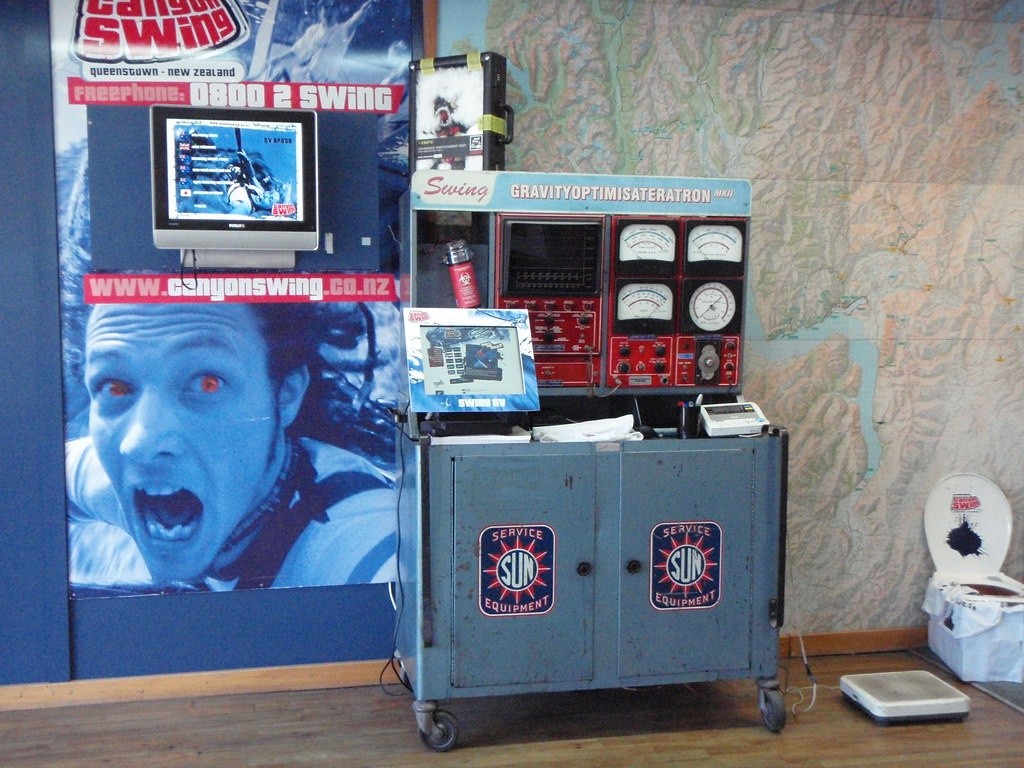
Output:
[[919, 570, 1024, 685]]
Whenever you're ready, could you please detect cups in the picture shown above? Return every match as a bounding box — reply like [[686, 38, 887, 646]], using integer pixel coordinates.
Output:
[[680, 407, 700, 439]]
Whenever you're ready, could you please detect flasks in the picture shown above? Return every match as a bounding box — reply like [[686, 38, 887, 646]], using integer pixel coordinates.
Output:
[[442, 238, 481, 308]]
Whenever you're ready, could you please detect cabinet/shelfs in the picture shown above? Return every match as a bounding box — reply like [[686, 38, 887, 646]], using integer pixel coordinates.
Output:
[[391, 385, 790, 753]]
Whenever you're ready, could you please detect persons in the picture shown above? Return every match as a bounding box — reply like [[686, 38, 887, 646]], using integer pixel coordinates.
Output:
[[174, 129, 293, 216], [62, 300, 404, 592]]
